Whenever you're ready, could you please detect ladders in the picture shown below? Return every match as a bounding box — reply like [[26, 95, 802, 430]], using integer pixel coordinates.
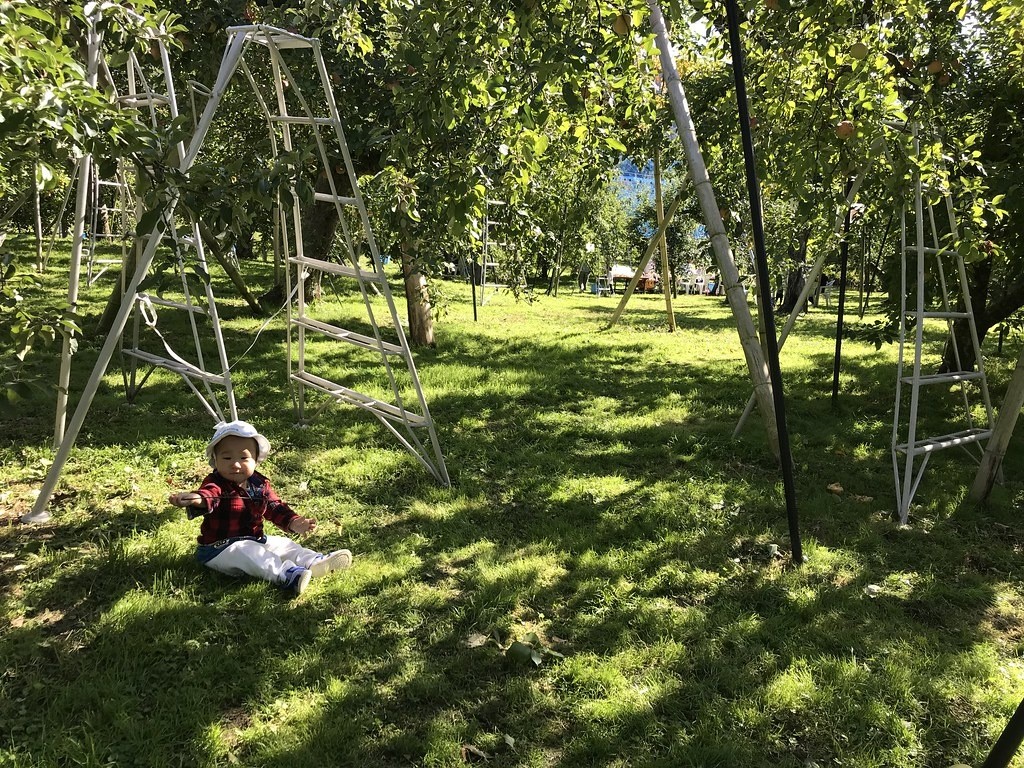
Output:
[[733, 88, 1005, 525], [480, 190, 533, 306], [596, 244, 612, 297], [16, 2, 453, 526]]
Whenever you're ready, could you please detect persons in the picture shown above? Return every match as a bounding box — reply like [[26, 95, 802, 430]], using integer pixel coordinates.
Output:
[[579, 263, 615, 295], [169, 421, 352, 593]]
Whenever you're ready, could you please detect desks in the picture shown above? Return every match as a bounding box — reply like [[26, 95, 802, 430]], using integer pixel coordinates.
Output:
[[610, 277, 649, 295]]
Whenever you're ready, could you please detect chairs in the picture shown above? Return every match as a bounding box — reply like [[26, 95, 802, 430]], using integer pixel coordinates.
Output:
[[597, 278, 611, 297], [816, 280, 835, 306], [680, 270, 706, 295]]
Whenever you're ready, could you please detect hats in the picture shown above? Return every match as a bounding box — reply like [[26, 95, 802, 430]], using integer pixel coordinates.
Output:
[[205, 420, 271, 465]]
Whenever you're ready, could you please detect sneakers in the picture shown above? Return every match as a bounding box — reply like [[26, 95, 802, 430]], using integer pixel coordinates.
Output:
[[280, 566, 312, 593], [308, 549, 352, 578]]
